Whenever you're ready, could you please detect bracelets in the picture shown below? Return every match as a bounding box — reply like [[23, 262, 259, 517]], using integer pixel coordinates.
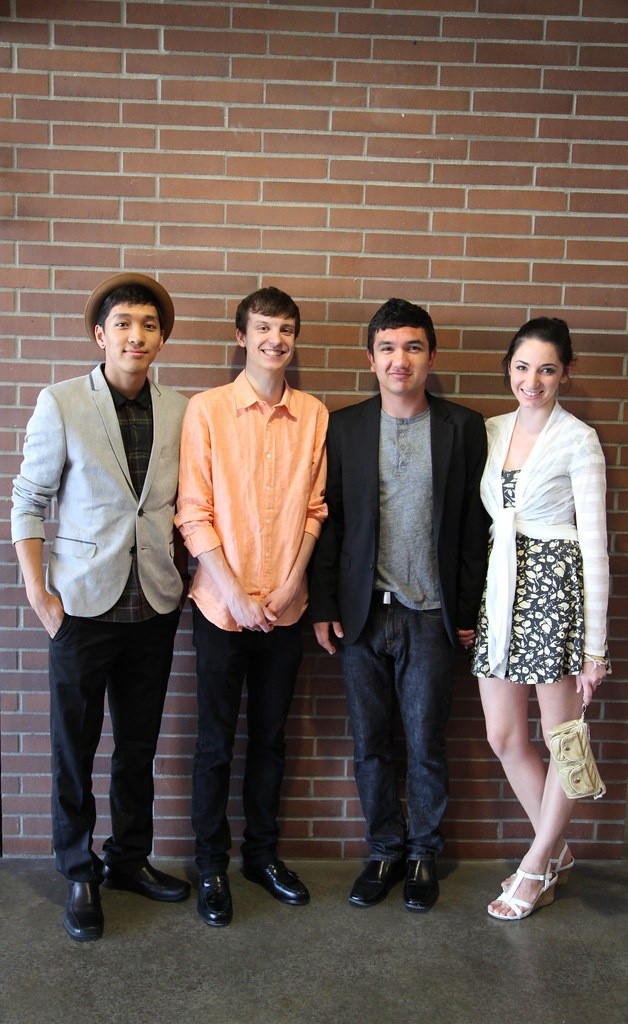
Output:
[[583, 655, 607, 669]]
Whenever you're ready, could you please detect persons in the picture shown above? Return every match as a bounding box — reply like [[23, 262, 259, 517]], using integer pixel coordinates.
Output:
[[10, 274, 191, 943], [312, 297, 493, 915], [458, 318, 615, 919], [170, 288, 328, 927]]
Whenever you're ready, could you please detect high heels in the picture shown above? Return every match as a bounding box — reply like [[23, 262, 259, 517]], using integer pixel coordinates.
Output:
[[501, 843, 575, 892], [487, 859, 558, 920]]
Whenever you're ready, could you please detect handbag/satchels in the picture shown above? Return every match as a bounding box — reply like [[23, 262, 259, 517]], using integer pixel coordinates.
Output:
[[548, 657, 607, 800]]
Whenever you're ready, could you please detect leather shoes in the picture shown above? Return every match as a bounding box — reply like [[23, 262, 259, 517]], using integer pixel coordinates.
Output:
[[348, 860, 406, 908], [63, 879, 104, 942], [403, 859, 440, 912], [243, 862, 310, 905], [102, 862, 191, 902], [197, 871, 233, 926]]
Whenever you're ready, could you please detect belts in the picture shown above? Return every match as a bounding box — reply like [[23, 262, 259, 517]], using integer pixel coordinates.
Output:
[[372, 591, 397, 606]]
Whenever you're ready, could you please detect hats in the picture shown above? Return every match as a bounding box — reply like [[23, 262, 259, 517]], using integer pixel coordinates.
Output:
[[84, 272, 175, 351]]
